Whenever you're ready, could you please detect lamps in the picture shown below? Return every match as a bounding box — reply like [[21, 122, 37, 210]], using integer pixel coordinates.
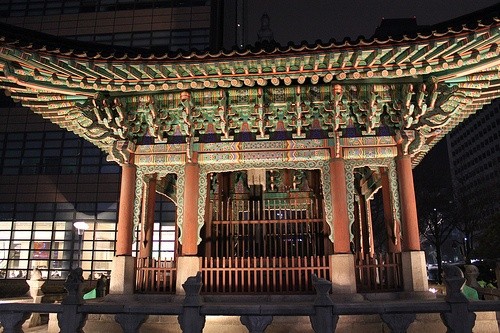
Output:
[[73, 222, 89, 267]]
